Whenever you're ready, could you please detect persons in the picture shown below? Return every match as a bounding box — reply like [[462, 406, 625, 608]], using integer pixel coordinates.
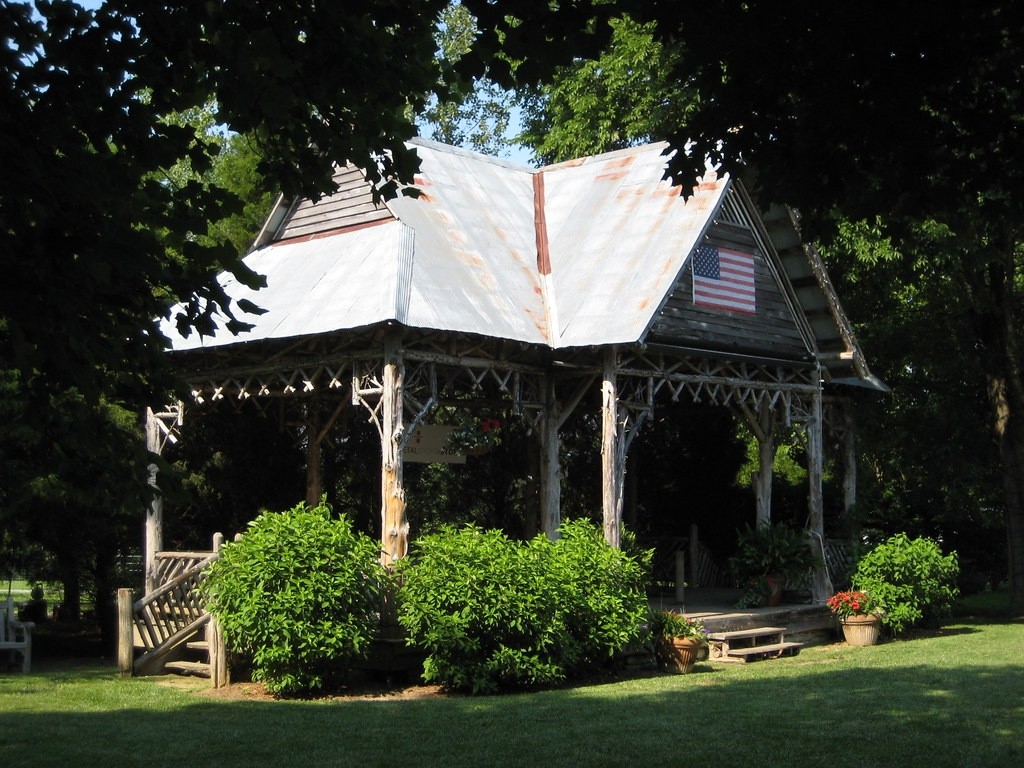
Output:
[[18, 588, 47, 623]]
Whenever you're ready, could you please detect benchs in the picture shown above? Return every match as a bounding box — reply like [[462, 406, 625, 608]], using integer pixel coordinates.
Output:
[[0, 596, 35, 675]]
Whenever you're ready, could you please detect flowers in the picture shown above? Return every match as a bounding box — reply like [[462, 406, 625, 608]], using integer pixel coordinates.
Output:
[[651, 608, 712, 648], [826, 591, 886, 622]]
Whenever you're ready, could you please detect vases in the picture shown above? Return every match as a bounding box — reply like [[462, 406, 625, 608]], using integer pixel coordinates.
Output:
[[659, 637, 704, 674], [839, 615, 882, 646]]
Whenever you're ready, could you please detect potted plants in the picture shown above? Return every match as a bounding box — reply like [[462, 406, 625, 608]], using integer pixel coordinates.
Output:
[[729, 515, 830, 611]]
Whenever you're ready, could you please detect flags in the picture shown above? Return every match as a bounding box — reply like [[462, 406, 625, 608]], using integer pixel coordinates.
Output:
[[692, 243, 756, 313]]
